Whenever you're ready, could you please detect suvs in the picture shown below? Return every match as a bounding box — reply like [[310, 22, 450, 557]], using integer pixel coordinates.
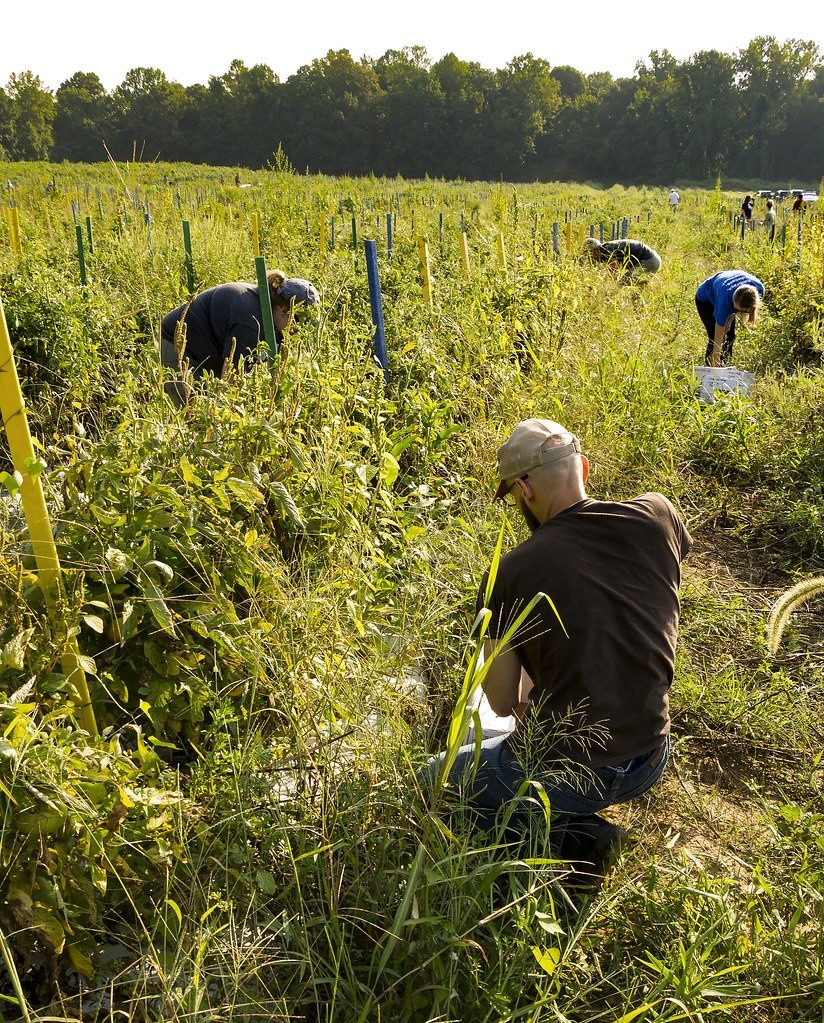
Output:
[[803, 193, 819, 204], [788, 188, 802, 198], [756, 189, 772, 199], [773, 189, 789, 203]]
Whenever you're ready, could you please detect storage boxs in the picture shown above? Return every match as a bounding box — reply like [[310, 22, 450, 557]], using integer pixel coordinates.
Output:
[[695, 366, 755, 404], [462, 645, 516, 747]]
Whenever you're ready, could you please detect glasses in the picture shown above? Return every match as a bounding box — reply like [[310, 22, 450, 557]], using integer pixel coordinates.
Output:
[[499, 474, 530, 507]]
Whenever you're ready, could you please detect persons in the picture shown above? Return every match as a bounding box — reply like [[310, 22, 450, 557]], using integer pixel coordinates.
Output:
[[161, 270, 320, 412], [417, 418, 694, 912], [759, 200, 776, 253], [581, 237, 661, 301], [741, 196, 755, 221], [669, 189, 679, 214], [695, 270, 765, 367], [792, 195, 807, 217]]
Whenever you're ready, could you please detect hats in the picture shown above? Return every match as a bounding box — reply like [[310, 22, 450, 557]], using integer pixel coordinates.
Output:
[[278, 278, 327, 328], [491, 418, 581, 504], [583, 237, 601, 252]]
